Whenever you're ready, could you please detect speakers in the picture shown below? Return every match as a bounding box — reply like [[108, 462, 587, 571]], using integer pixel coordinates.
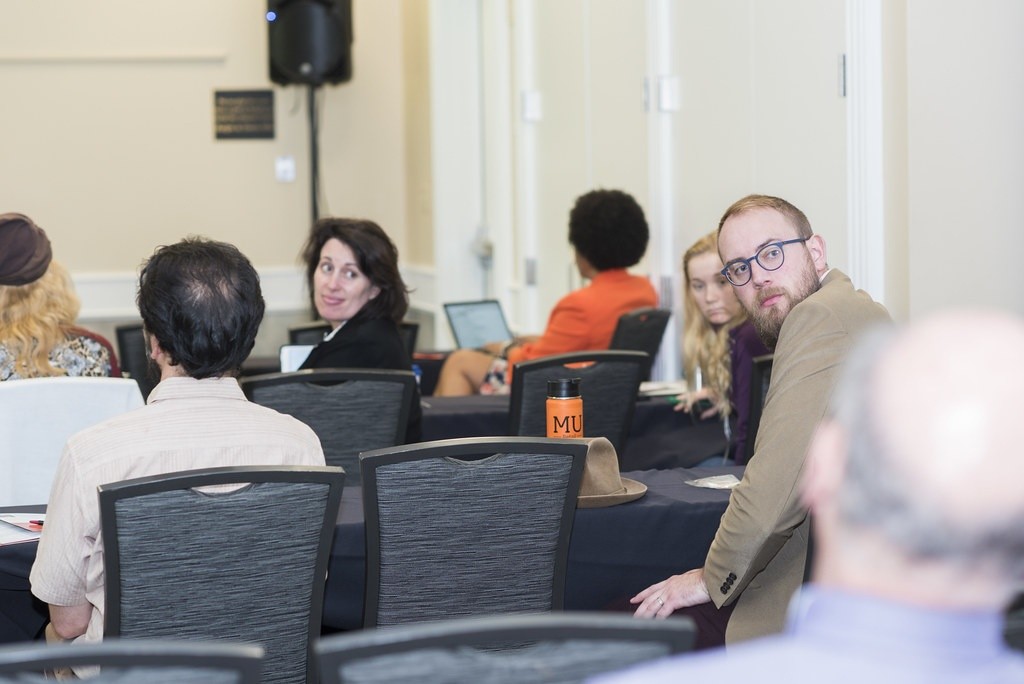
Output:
[[267, 0, 353, 88]]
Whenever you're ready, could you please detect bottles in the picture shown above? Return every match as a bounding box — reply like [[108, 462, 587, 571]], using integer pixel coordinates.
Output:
[[545, 377, 584, 438]]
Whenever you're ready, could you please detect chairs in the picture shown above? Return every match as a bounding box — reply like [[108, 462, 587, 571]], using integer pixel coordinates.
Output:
[[0, 307, 815, 684]]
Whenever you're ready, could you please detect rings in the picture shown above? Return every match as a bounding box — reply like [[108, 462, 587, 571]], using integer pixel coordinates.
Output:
[[657, 597, 664, 606]]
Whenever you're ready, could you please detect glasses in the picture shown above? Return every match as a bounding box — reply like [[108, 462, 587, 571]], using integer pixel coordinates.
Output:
[[720, 237, 809, 286]]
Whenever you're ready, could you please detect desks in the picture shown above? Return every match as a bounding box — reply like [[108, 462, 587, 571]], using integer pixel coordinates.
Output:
[[242, 348, 477, 440], [0, 467, 748, 684], [417, 388, 722, 458]]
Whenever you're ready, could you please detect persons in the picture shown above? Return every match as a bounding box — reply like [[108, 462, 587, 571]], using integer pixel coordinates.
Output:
[[430, 186, 660, 398], [293, 217, 424, 447], [0, 235, 325, 684], [669, 230, 775, 467], [0, 212, 111, 380], [630, 195, 892, 649], [592, 310, 1024, 684]]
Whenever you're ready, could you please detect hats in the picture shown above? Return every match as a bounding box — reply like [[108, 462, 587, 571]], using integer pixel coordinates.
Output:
[[0, 213, 52, 286], [576, 436, 648, 508]]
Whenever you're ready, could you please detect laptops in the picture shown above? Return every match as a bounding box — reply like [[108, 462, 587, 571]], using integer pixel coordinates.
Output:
[[444, 300, 513, 351]]
